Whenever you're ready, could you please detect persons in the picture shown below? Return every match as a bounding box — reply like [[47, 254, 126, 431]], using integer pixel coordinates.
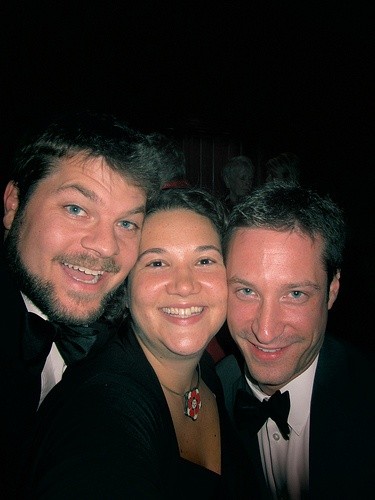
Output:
[[216, 168, 375, 500], [0, 120, 177, 500], [33, 180, 232, 500], [222, 155, 255, 213], [154, 136, 192, 188]]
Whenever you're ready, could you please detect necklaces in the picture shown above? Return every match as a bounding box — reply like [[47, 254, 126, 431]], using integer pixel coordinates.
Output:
[[167, 364, 202, 421]]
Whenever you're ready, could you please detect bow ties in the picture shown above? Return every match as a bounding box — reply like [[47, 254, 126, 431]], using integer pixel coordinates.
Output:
[[235, 388, 291, 441], [21, 311, 98, 369]]
